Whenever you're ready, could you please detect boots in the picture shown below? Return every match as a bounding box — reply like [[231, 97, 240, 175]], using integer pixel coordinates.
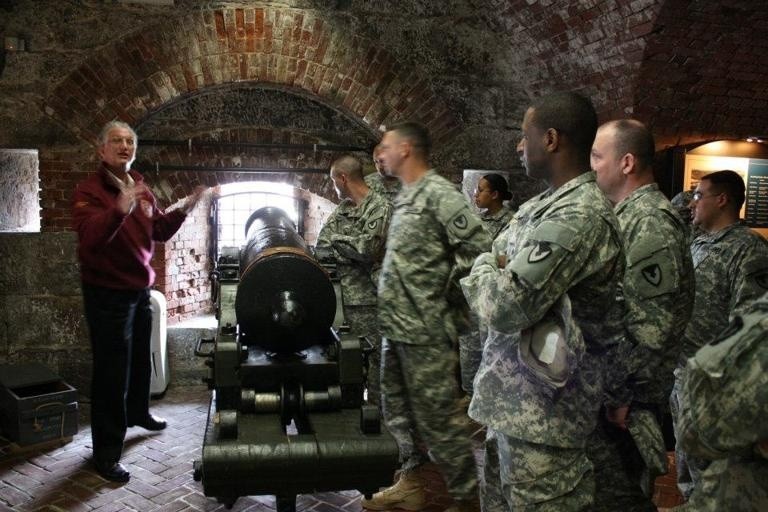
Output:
[[360, 470, 424, 510]]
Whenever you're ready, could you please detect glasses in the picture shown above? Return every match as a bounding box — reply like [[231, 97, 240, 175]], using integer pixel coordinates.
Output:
[[693, 192, 715, 201]]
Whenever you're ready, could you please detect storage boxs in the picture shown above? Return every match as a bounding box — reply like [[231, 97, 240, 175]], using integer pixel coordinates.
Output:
[[0, 362, 82, 448]]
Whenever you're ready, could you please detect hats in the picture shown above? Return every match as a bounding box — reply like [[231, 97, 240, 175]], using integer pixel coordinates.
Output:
[[626, 410, 669, 476], [518, 293, 586, 390]]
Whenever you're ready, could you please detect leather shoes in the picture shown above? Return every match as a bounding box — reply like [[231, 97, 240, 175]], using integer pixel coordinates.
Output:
[[128, 414, 166, 430], [95, 462, 129, 482]]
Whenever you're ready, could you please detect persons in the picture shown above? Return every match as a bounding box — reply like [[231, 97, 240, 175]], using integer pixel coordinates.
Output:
[[451, 170, 514, 415], [359, 120, 496, 511], [362, 144, 404, 218], [670, 169, 768, 512], [590, 115, 699, 512], [70, 119, 222, 484], [309, 154, 394, 417], [452, 88, 631, 511], [667, 292, 768, 512]]
